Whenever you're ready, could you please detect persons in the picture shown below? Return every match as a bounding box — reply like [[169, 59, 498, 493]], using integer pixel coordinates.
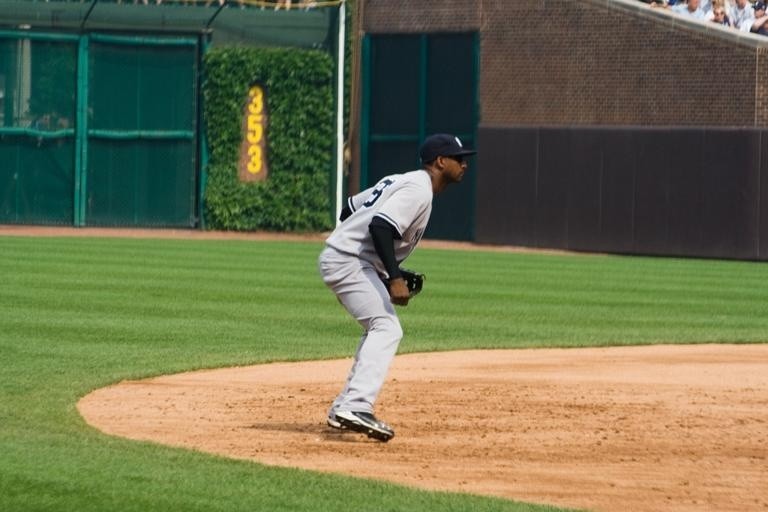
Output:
[[319, 133, 478, 444], [639, 0, 768, 39]]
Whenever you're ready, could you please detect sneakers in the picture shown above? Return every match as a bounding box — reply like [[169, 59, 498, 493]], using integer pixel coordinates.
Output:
[[336, 407, 395, 442], [326, 413, 362, 432]]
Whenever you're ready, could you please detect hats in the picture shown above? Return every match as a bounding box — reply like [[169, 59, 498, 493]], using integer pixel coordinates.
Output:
[[751, 0, 767, 10], [421, 132, 477, 162]]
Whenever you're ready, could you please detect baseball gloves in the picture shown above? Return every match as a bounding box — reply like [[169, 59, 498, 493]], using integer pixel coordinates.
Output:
[[384, 267, 423, 300]]
